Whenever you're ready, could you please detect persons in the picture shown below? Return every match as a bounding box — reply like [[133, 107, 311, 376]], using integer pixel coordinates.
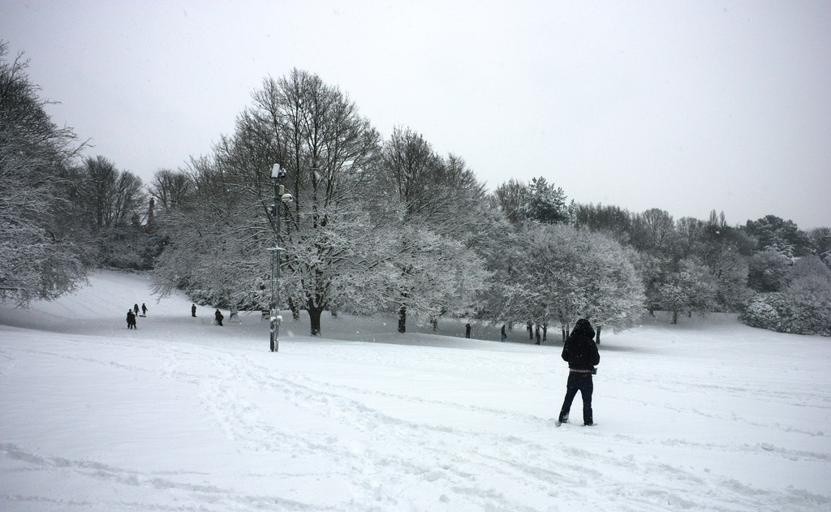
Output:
[[125, 308, 132, 329], [216, 311, 224, 326], [214, 309, 219, 320], [557, 319, 601, 425], [191, 304, 196, 317], [141, 303, 147, 316], [133, 304, 139, 315], [129, 312, 136, 328]]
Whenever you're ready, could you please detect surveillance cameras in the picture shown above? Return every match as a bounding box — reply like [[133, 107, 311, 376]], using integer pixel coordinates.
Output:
[[271, 163, 280, 179]]
